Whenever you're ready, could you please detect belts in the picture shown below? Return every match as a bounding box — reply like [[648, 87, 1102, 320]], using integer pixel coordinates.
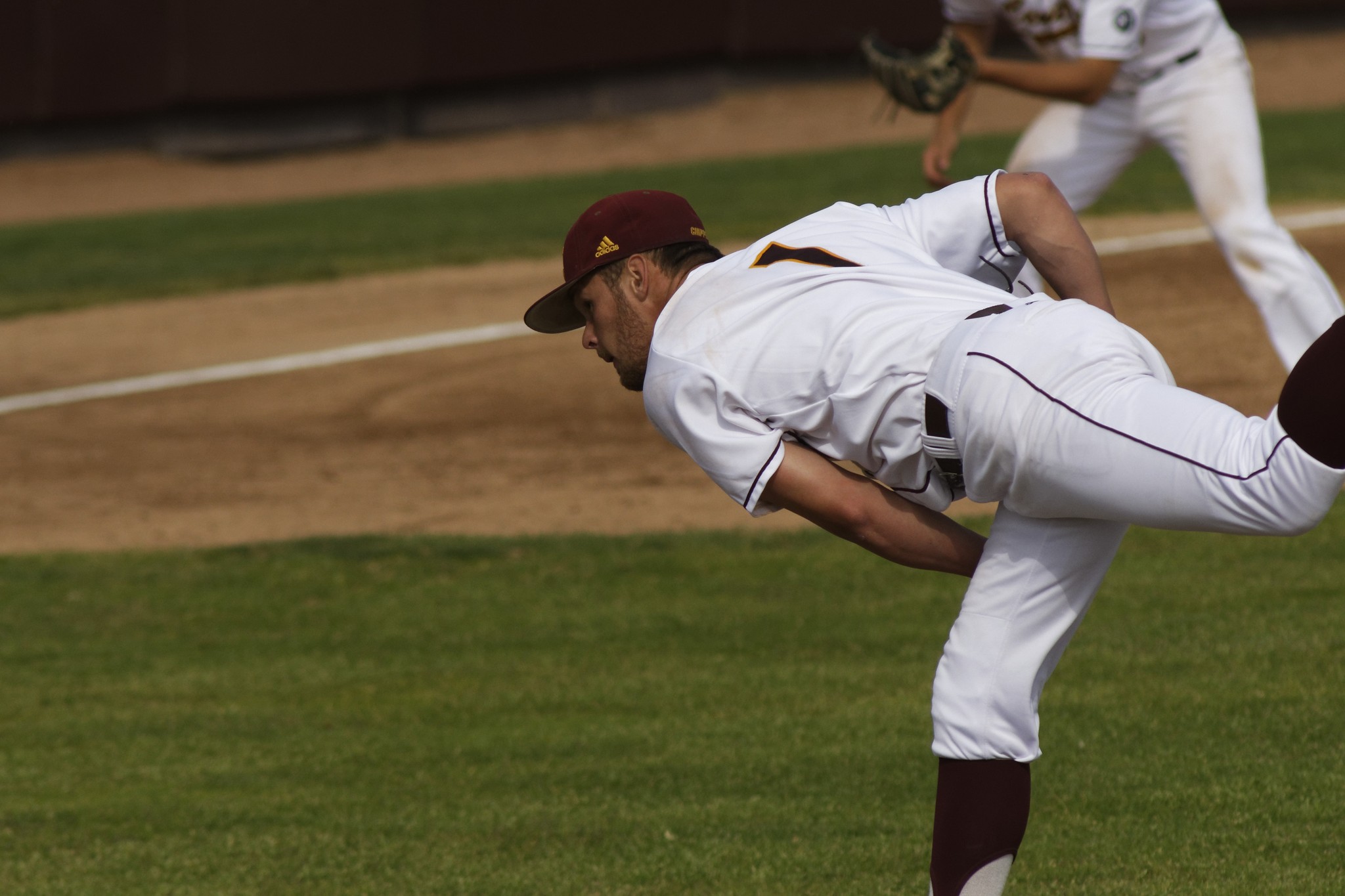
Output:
[[925, 301, 1037, 502], [1178, 51, 1198, 62]]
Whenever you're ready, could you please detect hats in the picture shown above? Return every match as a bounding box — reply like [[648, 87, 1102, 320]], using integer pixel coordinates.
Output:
[[524, 189, 710, 333]]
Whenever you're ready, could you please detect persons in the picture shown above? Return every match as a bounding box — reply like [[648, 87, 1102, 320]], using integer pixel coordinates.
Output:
[[522, 168, 1345, 896], [919, 1, 1345, 376]]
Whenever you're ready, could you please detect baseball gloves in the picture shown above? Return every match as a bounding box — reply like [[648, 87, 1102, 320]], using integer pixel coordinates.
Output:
[[860, 25, 973, 114]]
